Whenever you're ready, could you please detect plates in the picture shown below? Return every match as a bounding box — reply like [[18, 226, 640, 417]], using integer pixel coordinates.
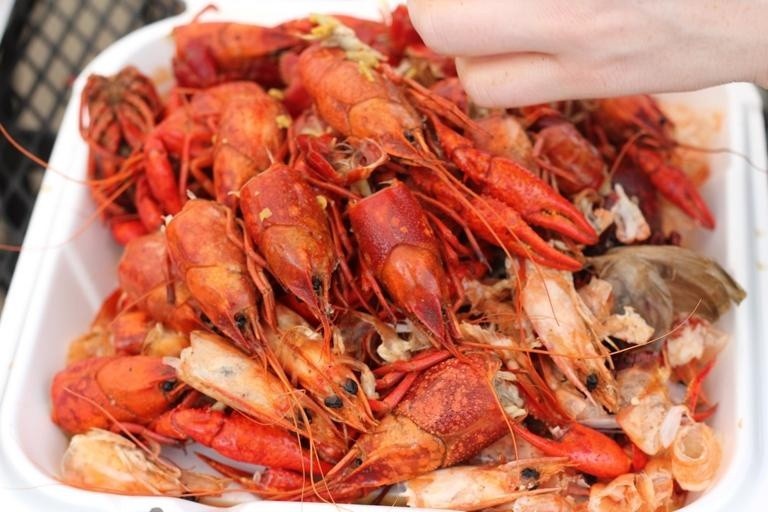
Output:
[[0, 3, 768, 509]]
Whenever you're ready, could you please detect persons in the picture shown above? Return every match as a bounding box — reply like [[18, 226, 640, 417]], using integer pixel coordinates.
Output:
[[407, 0, 768, 109]]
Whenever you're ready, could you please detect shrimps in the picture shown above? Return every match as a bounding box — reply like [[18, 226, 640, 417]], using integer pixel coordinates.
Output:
[[51, 5, 748, 511]]
